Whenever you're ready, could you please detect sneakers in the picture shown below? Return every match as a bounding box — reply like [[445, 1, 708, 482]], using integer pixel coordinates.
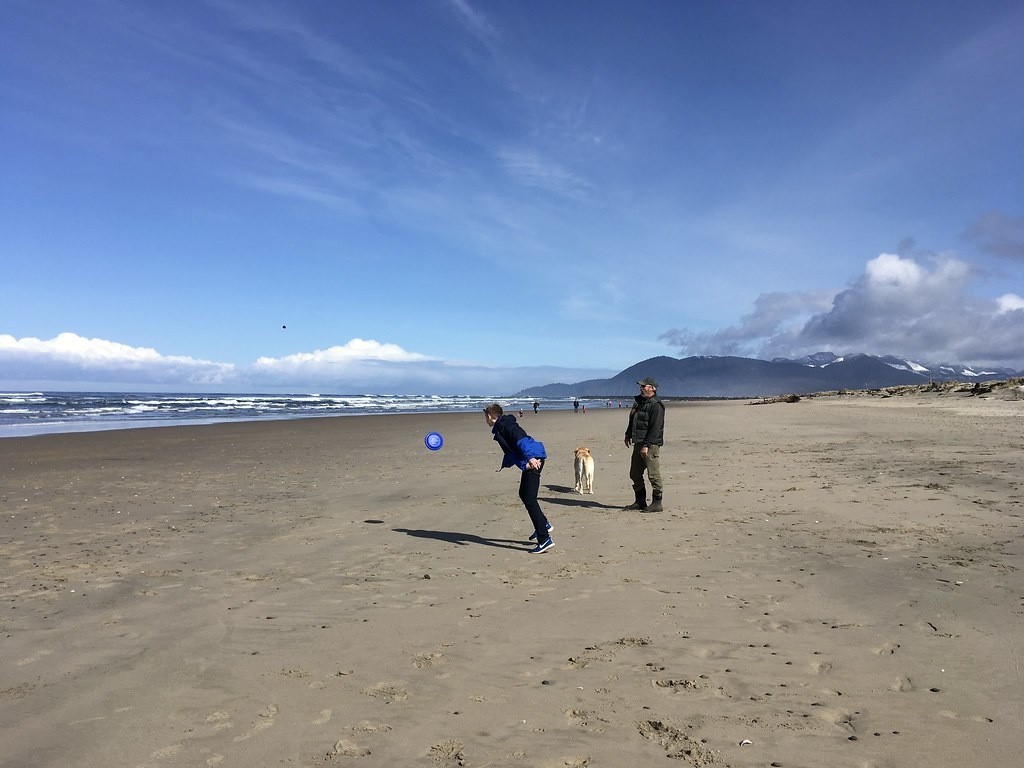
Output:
[[529, 521, 554, 540], [530, 537, 555, 554]]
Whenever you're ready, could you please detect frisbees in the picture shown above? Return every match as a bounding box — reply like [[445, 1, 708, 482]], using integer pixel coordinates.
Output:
[[424, 431, 443, 453]]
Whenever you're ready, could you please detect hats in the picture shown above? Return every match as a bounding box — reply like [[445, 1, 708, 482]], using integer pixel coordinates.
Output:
[[636, 377, 659, 388]]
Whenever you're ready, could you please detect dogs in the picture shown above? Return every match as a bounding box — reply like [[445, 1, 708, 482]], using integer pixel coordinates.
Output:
[[574, 447, 594, 495]]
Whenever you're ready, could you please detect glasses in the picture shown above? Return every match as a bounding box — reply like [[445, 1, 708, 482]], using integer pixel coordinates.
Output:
[[483, 406, 489, 414]]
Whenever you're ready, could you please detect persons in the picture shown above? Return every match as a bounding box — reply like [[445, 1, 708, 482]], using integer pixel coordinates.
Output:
[[482, 404, 555, 554], [624, 377, 667, 513], [519, 398, 622, 419]]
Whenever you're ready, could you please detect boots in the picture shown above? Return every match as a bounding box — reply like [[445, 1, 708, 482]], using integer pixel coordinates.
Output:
[[625, 490, 647, 509], [643, 492, 663, 512]]
